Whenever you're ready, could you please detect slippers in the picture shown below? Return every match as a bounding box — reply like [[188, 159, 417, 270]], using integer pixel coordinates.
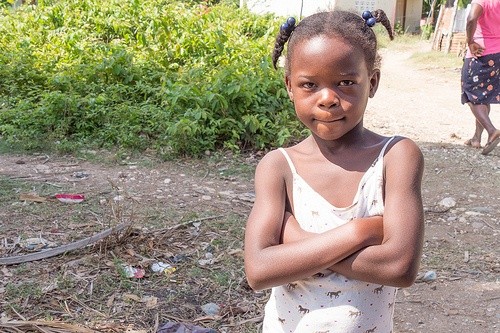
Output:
[[464, 139, 481, 149], [482, 136, 500, 155]]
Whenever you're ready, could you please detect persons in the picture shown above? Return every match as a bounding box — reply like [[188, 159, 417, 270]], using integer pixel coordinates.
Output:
[[243, 7, 424, 333], [458, 0, 500, 155]]
